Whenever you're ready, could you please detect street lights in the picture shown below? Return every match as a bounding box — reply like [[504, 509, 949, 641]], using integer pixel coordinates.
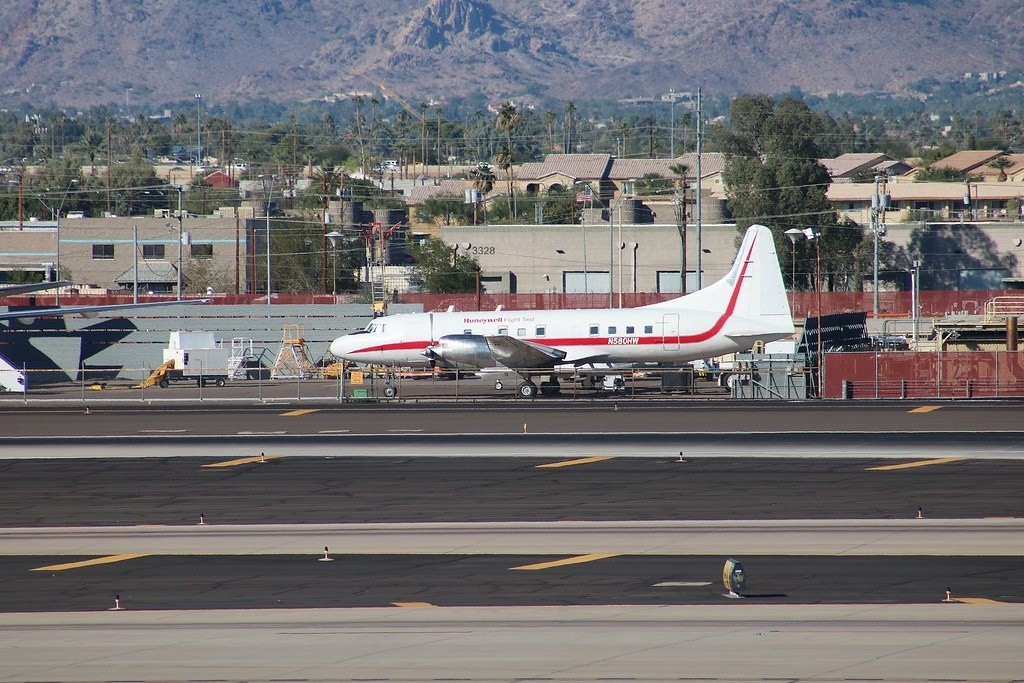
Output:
[[55, 180, 79, 306], [258, 174, 278, 305], [782, 227, 804, 322], [324, 232, 345, 294], [194, 93, 203, 166]]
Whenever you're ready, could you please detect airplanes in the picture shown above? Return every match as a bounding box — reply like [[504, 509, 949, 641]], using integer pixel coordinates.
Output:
[[329, 224, 797, 399]]
